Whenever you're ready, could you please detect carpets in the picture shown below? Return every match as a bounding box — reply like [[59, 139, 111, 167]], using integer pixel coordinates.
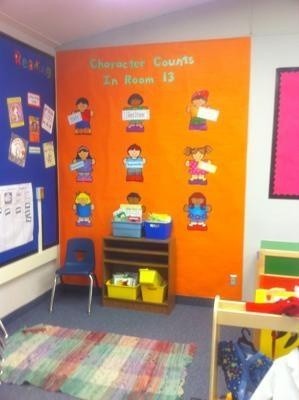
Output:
[[1, 323, 196, 400]]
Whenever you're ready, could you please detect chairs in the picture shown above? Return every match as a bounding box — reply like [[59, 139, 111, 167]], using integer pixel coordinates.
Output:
[[50, 237, 101, 315]]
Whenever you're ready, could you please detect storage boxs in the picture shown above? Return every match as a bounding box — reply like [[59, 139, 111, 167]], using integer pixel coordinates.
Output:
[[144, 220, 172, 240], [105, 278, 141, 300], [112, 221, 143, 238], [140, 284, 167, 304], [138, 268, 163, 285]]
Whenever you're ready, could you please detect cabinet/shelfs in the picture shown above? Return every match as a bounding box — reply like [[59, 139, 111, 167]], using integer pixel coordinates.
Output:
[[101, 235, 177, 314]]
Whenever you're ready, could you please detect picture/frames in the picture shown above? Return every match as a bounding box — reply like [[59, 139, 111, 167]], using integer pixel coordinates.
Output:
[[268, 67, 299, 199]]
